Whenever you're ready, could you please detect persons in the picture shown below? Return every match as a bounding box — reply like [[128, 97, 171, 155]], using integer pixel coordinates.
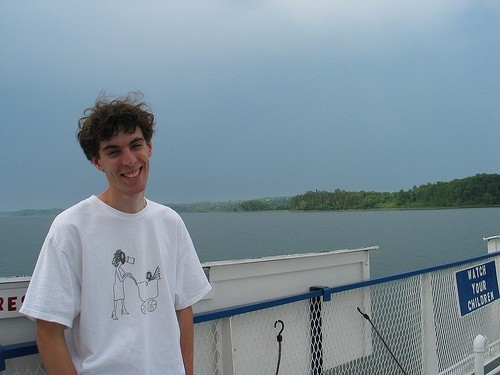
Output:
[[16, 87, 213, 375]]
